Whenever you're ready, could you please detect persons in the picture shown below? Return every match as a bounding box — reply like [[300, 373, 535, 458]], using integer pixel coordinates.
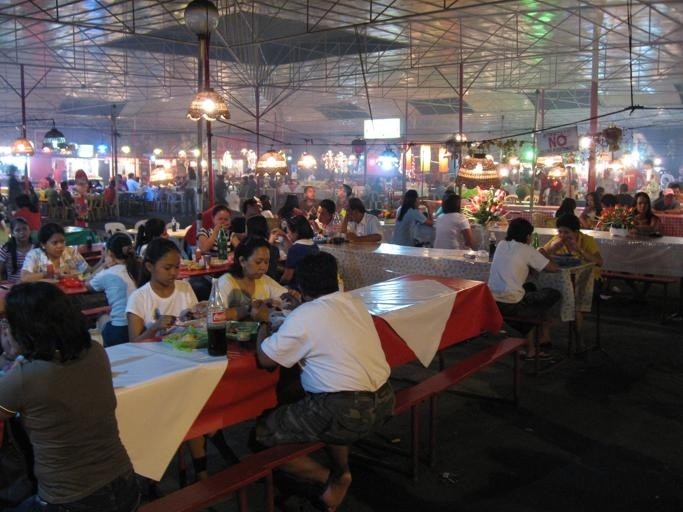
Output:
[[1, 162, 683, 364], [0, 280, 141, 510], [256, 251, 397, 511], [125, 239, 238, 479]]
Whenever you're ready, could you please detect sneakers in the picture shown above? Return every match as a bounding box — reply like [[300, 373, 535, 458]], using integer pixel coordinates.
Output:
[[538, 354, 553, 361], [525, 354, 537, 361], [540, 336, 551, 344]]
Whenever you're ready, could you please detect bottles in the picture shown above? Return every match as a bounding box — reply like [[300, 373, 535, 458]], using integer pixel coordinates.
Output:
[[170, 214, 177, 234], [487, 231, 497, 264], [216, 224, 228, 261], [45, 259, 53, 279], [204, 277, 227, 357], [331, 213, 343, 247], [532, 232, 539, 249]]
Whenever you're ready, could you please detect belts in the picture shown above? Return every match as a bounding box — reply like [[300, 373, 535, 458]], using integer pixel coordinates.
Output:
[[330, 383, 389, 398]]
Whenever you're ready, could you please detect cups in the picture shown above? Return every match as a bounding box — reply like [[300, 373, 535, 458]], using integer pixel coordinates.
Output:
[[203, 255, 212, 270]]
